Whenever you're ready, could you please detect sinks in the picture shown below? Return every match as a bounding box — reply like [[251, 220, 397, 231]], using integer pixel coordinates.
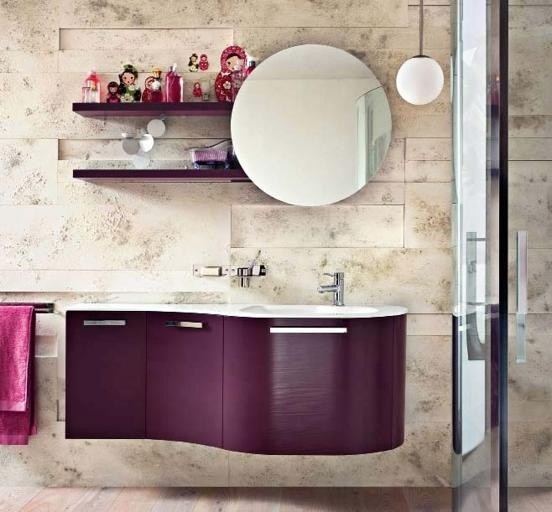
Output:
[[240, 305, 380, 317]]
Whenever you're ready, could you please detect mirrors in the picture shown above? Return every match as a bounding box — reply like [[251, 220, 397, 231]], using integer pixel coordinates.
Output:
[[231, 42, 394, 209]]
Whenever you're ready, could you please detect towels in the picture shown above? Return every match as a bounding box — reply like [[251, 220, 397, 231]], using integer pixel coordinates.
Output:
[[0, 305, 35, 447]]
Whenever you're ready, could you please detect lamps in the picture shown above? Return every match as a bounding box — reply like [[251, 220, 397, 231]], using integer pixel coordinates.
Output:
[[392, 2, 443, 106]]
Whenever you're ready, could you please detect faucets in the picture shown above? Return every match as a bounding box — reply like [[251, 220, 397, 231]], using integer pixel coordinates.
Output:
[[318, 271, 344, 306]]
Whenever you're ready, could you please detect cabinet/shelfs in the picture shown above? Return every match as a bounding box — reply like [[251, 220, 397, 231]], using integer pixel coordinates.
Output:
[[219, 315, 406, 454], [144, 313, 222, 449], [65, 311, 145, 439], [70, 100, 247, 182]]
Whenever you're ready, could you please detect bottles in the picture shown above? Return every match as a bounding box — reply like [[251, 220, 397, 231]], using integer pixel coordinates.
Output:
[[81, 69, 102, 104], [165, 64, 184, 103]]
